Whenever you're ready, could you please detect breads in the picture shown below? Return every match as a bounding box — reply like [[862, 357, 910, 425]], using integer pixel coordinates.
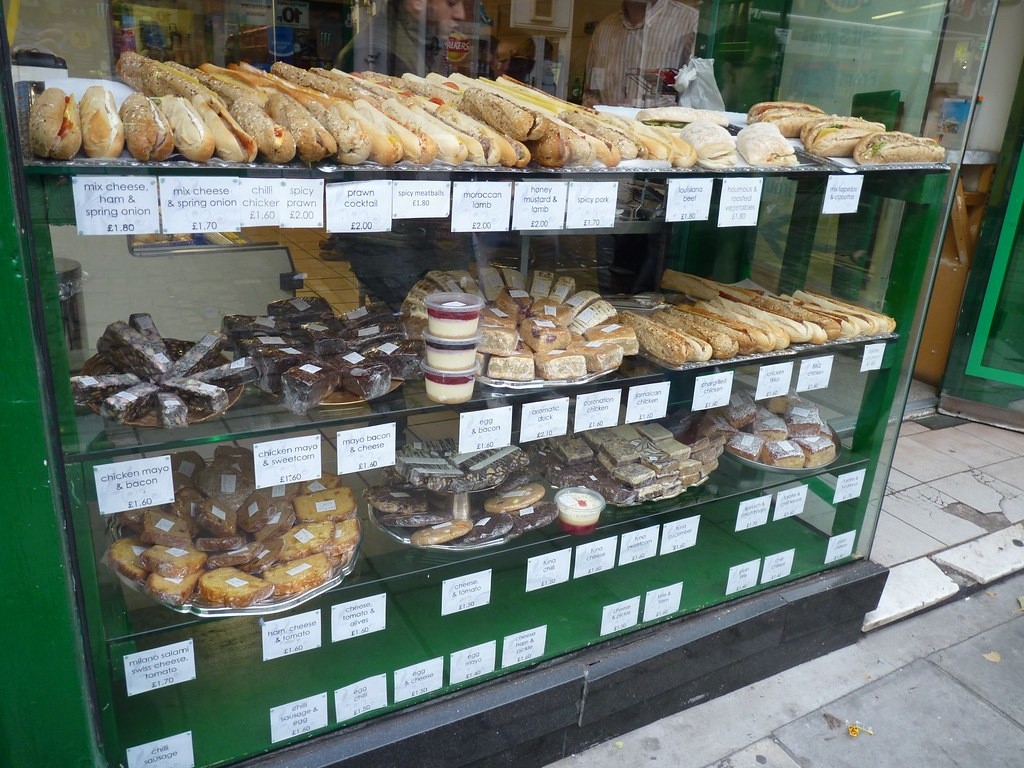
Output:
[[68, 261, 898, 612]]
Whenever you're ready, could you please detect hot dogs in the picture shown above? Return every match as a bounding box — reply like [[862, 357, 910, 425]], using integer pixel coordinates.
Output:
[[26, 50, 946, 167]]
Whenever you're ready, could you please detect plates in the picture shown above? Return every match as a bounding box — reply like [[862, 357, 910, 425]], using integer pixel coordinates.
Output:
[[713, 421, 843, 474], [540, 466, 709, 507], [251, 369, 406, 405], [80, 337, 245, 427], [367, 500, 525, 552], [103, 512, 366, 617], [475, 364, 620, 392]]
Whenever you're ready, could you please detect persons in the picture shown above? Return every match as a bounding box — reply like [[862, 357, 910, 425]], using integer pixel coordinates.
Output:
[[581, 1, 703, 108], [490, 29, 536, 87], [336, 0, 473, 305]]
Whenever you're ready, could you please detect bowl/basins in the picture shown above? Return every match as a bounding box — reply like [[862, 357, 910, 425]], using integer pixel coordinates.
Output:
[[423, 291, 485, 340], [420, 356, 481, 404], [421, 326, 483, 370], [553, 487, 606, 537]]
